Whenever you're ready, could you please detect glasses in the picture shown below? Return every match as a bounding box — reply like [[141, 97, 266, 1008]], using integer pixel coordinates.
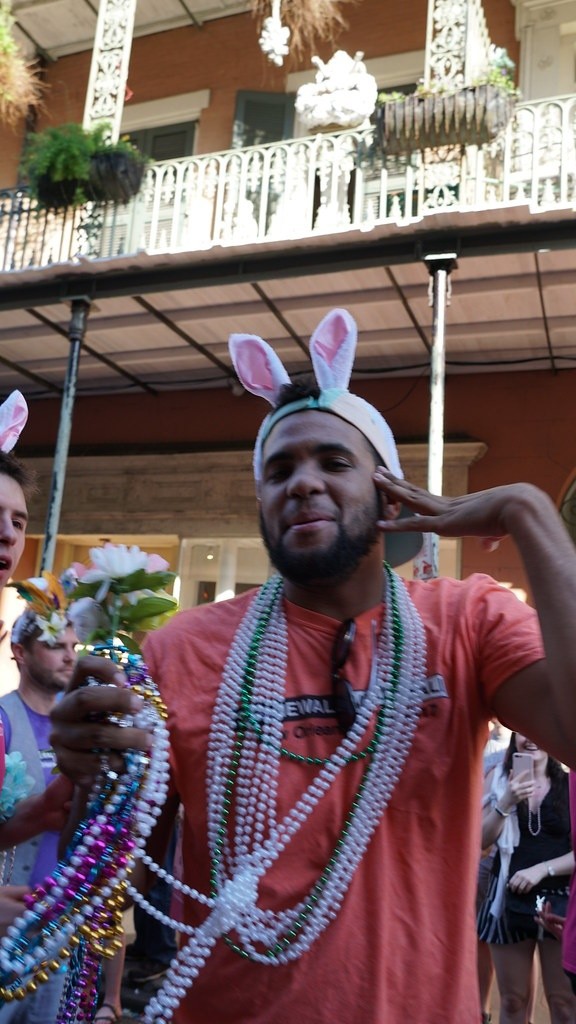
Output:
[[330, 618, 356, 737]]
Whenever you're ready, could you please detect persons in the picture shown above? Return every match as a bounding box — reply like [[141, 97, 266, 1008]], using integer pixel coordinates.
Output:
[[0, 607, 81, 1024], [0, 451, 39, 592], [88, 894, 132, 1024], [479, 714, 576, 1024], [124, 800, 187, 983], [48, 378, 576, 1024]]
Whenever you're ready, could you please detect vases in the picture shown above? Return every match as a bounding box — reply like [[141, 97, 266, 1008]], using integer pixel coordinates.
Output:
[[376, 83, 517, 156]]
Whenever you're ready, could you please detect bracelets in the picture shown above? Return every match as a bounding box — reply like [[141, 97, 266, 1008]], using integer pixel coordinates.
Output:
[[494, 805, 509, 817], [546, 863, 555, 876]]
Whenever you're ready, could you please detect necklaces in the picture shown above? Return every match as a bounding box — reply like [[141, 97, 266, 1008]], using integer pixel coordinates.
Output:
[[528, 795, 541, 836], [0, 560, 428, 1024]]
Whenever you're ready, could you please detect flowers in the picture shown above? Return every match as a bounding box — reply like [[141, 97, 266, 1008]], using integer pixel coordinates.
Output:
[[66, 543, 180, 666], [33, 612, 69, 649], [375, 66, 524, 105]]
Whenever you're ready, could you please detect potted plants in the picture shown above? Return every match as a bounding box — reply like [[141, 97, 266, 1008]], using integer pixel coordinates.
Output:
[[13, 121, 156, 219]]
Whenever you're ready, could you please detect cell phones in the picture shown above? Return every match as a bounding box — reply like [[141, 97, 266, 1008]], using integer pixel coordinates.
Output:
[[513, 753, 534, 794]]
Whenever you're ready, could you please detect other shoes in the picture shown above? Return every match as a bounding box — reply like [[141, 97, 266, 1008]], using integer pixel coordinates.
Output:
[[126, 943, 159, 956], [127, 960, 168, 982], [91, 1003, 123, 1024]]
